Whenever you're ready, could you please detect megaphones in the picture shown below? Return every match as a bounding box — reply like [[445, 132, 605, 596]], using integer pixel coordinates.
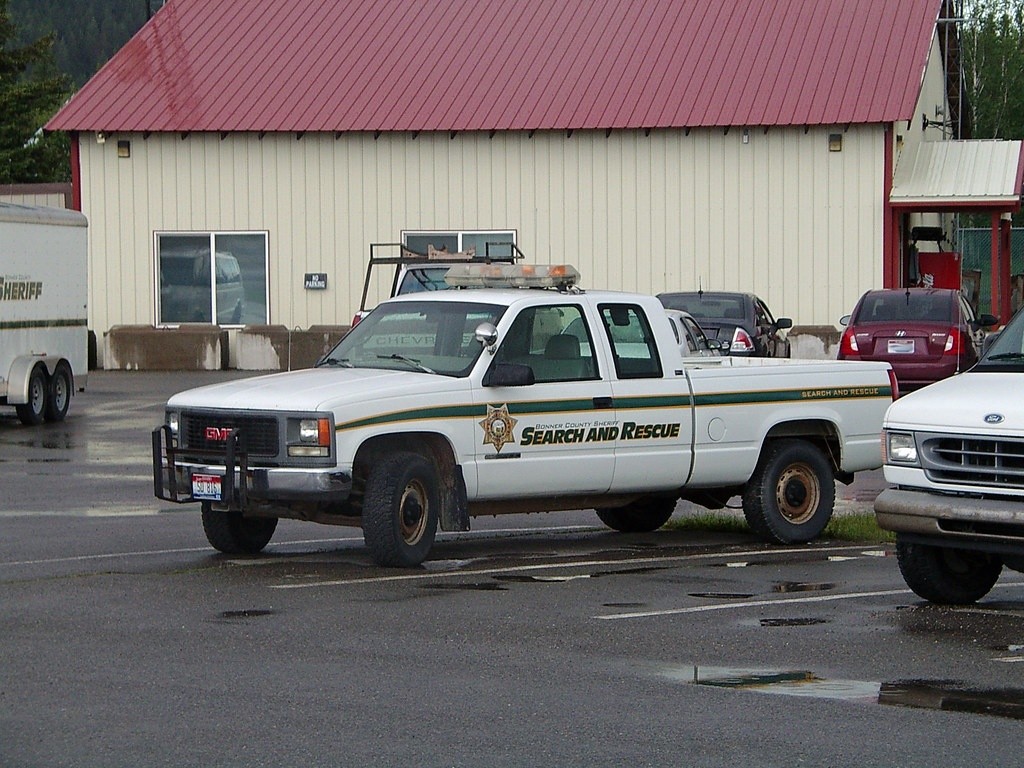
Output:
[[94, 131, 105, 143]]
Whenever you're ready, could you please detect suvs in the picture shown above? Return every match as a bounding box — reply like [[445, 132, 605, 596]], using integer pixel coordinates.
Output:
[[873, 299, 1024, 605]]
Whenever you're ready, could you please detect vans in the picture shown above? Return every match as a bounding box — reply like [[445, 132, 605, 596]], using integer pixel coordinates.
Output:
[[160, 246, 246, 324]]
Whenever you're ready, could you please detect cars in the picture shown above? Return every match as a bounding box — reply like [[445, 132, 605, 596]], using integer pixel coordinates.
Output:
[[653, 291, 793, 358], [560, 310, 722, 359], [836, 286, 999, 393]]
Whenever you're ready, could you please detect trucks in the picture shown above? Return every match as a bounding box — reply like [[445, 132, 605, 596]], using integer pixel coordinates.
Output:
[[1, 202, 89, 426]]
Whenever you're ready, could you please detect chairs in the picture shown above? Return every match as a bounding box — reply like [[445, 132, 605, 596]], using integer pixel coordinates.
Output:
[[724, 306, 742, 318], [874, 305, 897, 320], [516, 334, 589, 381], [670, 305, 688, 311]]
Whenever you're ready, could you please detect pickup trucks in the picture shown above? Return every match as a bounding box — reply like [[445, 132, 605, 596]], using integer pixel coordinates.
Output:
[[352, 264, 565, 361], [151, 261, 905, 570]]
